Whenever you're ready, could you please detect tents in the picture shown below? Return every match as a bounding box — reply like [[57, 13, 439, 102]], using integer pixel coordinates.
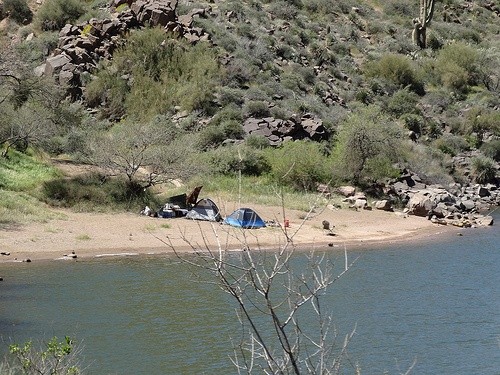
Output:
[[224, 208, 268, 229], [186, 199, 223, 223]]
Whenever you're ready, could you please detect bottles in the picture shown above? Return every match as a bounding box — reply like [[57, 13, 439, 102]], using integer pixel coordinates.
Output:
[[285, 220, 289, 227]]
